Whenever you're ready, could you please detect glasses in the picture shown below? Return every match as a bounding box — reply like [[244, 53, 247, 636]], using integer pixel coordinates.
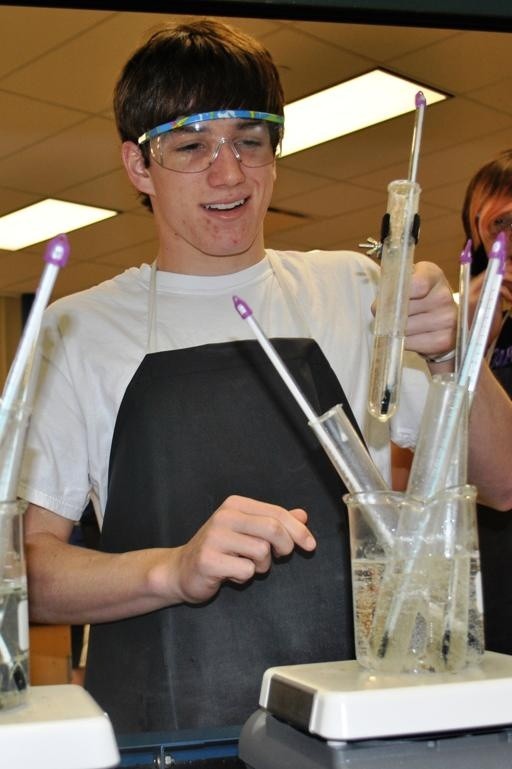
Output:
[[145, 124, 283, 174]]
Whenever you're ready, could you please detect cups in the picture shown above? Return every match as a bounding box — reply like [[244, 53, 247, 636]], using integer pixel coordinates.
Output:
[[343, 485, 484, 675], [0, 498, 31, 712]]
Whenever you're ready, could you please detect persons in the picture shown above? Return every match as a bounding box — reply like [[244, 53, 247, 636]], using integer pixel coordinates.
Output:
[[16, 20, 508, 760], [460, 145, 512, 657]]
